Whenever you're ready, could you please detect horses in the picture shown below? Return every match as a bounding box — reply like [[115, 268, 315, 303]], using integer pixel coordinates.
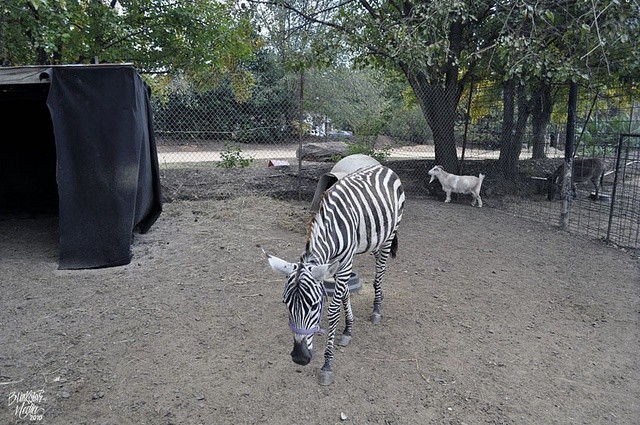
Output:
[[547, 158, 607, 202]]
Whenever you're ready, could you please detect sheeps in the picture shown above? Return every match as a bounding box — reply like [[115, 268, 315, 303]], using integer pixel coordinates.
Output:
[[427, 164, 486, 208]]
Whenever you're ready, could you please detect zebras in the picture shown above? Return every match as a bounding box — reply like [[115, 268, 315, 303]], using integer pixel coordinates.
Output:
[[261, 163, 406, 387]]
